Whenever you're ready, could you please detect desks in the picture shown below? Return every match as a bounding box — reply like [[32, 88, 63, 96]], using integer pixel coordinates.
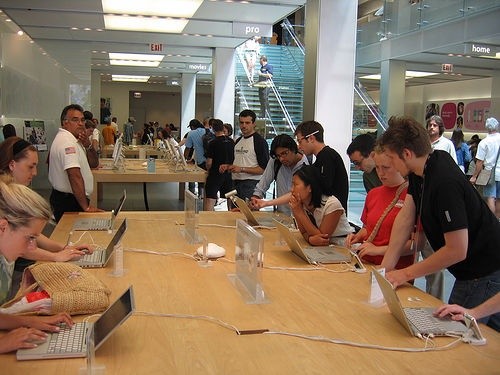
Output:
[[102, 144, 158, 158], [92, 158, 208, 208], [0, 210, 500, 375]]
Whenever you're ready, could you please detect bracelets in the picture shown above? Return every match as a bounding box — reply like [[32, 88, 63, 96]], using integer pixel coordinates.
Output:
[[240, 167, 244, 172], [85, 207, 89, 211]]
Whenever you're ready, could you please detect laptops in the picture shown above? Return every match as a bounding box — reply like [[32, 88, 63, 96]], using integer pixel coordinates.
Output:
[[98, 133, 126, 169], [163, 140, 198, 172], [371, 265, 468, 340], [57, 217, 127, 268], [71, 189, 126, 231], [16, 283, 134, 361], [235, 195, 288, 228], [271, 218, 352, 264]]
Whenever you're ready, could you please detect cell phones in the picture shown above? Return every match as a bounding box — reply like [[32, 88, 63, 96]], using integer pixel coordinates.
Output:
[[353, 253, 365, 269], [464, 313, 483, 340]]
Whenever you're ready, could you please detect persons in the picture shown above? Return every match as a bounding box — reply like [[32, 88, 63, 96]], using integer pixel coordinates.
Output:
[[3, 124, 16, 140], [178, 115, 234, 212], [251, 121, 349, 225], [255, 54, 273, 118], [351, 135, 425, 286], [426, 115, 500, 220], [218, 109, 270, 212], [433, 292, 500, 323], [248, 134, 310, 216], [121, 116, 180, 145], [47, 104, 104, 226], [0, 137, 93, 299], [289, 165, 357, 247], [157, 130, 180, 160], [102, 117, 120, 146], [82, 110, 103, 159], [377, 115, 500, 333], [0, 172, 75, 354], [346, 134, 445, 302]]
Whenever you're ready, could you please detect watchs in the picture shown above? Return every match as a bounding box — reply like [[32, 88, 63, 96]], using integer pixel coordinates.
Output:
[[85, 144, 92, 151]]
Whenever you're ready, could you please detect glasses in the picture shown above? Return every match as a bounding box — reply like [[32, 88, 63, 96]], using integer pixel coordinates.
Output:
[[274, 149, 290, 159], [354, 157, 365, 165], [65, 117, 86, 123]]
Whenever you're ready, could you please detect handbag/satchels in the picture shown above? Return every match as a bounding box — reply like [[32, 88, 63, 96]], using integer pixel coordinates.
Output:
[[0, 261, 108, 315], [473, 167, 495, 185]]
